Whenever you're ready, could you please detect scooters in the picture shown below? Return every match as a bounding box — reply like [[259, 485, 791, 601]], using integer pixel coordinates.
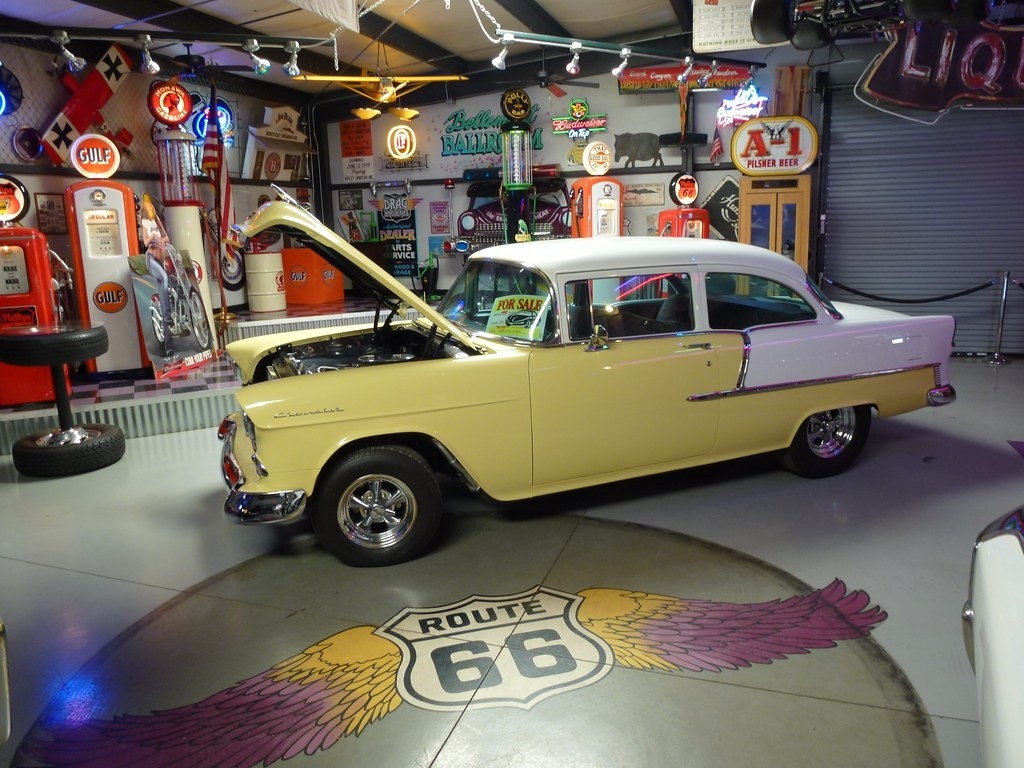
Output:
[[149, 239, 212, 351]]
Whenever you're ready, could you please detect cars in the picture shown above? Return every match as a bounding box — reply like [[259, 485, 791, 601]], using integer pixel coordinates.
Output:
[[962, 504, 1023, 768], [219, 181, 958, 568]]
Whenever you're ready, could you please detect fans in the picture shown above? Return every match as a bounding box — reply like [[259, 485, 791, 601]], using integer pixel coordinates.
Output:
[[147, 43, 256, 78]]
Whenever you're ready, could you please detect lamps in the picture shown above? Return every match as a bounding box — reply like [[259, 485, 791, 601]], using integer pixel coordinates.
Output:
[[491, 33, 517, 70], [282, 40, 302, 77], [612, 47, 632, 79], [242, 38, 271, 75], [696, 60, 720, 88], [739, 65, 757, 90], [565, 42, 583, 75], [675, 56, 695, 84], [49, 28, 87, 76], [132, 32, 161, 75]]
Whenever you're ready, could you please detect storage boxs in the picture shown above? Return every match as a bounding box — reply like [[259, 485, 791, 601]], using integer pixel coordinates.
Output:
[[280, 247, 345, 307]]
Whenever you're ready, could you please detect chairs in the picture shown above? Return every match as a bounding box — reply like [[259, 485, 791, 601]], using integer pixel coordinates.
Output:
[[656, 293, 691, 325]]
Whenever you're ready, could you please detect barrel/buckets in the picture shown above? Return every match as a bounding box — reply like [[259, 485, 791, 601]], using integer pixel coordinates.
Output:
[[243, 250, 286, 313]]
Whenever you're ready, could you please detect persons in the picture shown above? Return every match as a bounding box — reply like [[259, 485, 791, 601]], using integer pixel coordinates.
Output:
[[141, 193, 175, 358]]
